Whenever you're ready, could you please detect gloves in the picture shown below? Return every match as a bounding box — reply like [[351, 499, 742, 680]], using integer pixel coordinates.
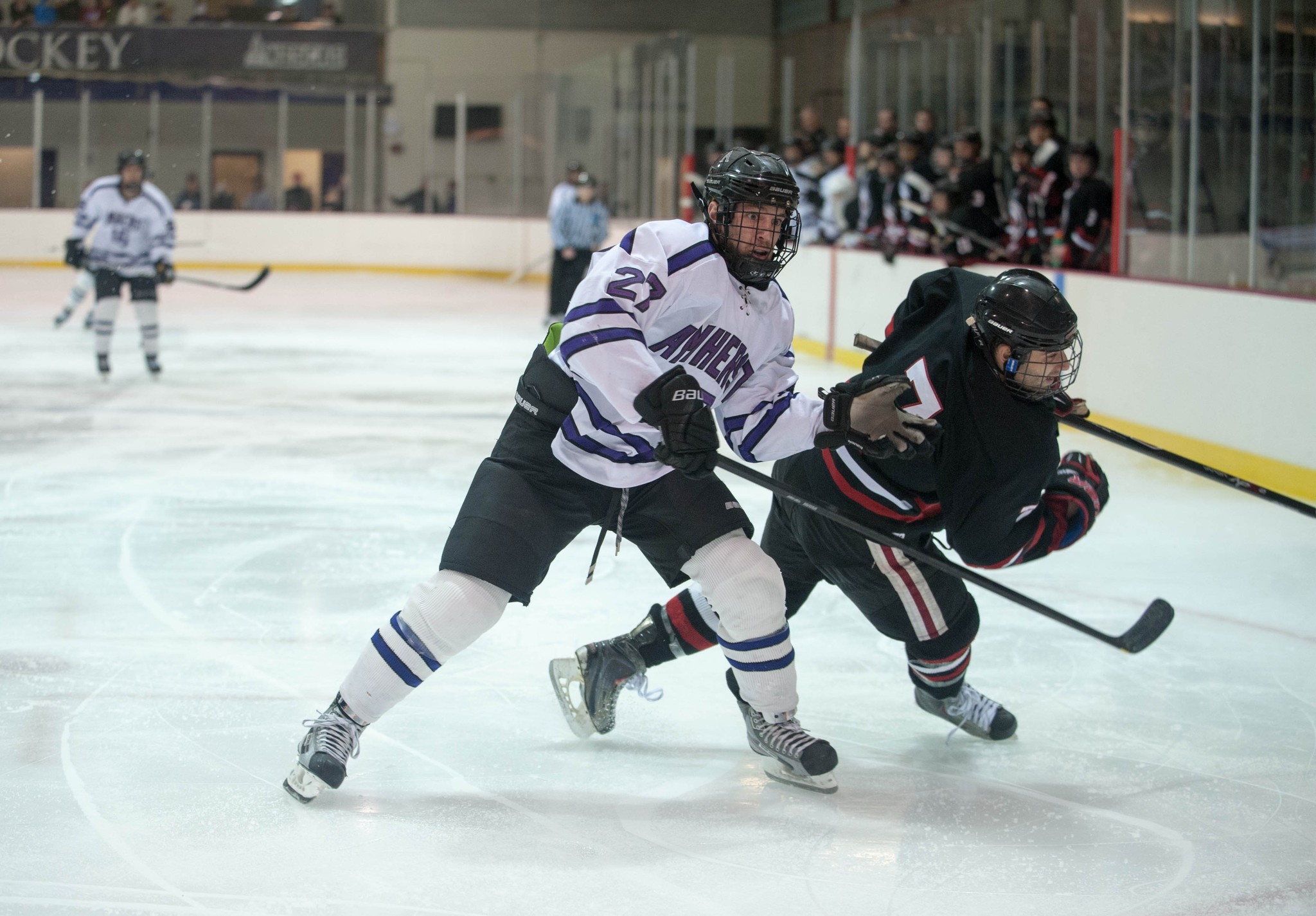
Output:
[[633, 364, 719, 481], [814, 374, 943, 461], [154, 258, 174, 287], [1040, 451, 1109, 550], [1051, 376, 1090, 418], [65, 239, 85, 268]]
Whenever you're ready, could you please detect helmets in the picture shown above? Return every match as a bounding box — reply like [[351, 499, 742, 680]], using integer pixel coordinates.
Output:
[[782, 112, 1099, 220], [704, 147, 802, 284], [972, 268, 1083, 402], [116, 148, 148, 189]]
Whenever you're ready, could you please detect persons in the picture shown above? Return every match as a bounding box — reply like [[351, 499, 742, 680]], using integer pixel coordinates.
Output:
[[542, 160, 611, 328], [550, 268, 1110, 750], [51, 154, 456, 380], [283, 145, 936, 805], [681, 97, 1107, 273]]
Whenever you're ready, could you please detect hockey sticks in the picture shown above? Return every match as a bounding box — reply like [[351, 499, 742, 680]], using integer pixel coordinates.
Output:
[[656, 443, 1175, 654], [898, 198, 1006, 255], [853, 332, 1316, 518], [82, 257, 269, 290]]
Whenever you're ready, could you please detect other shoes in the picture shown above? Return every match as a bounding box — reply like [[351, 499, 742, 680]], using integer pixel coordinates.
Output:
[[85, 313, 94, 328], [54, 308, 71, 324], [147, 355, 160, 372], [99, 355, 110, 373]]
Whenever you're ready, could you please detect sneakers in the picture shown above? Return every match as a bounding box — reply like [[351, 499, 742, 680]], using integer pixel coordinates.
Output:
[[725, 667, 839, 795], [914, 681, 1019, 747], [549, 603, 670, 738], [282, 690, 371, 804]]
